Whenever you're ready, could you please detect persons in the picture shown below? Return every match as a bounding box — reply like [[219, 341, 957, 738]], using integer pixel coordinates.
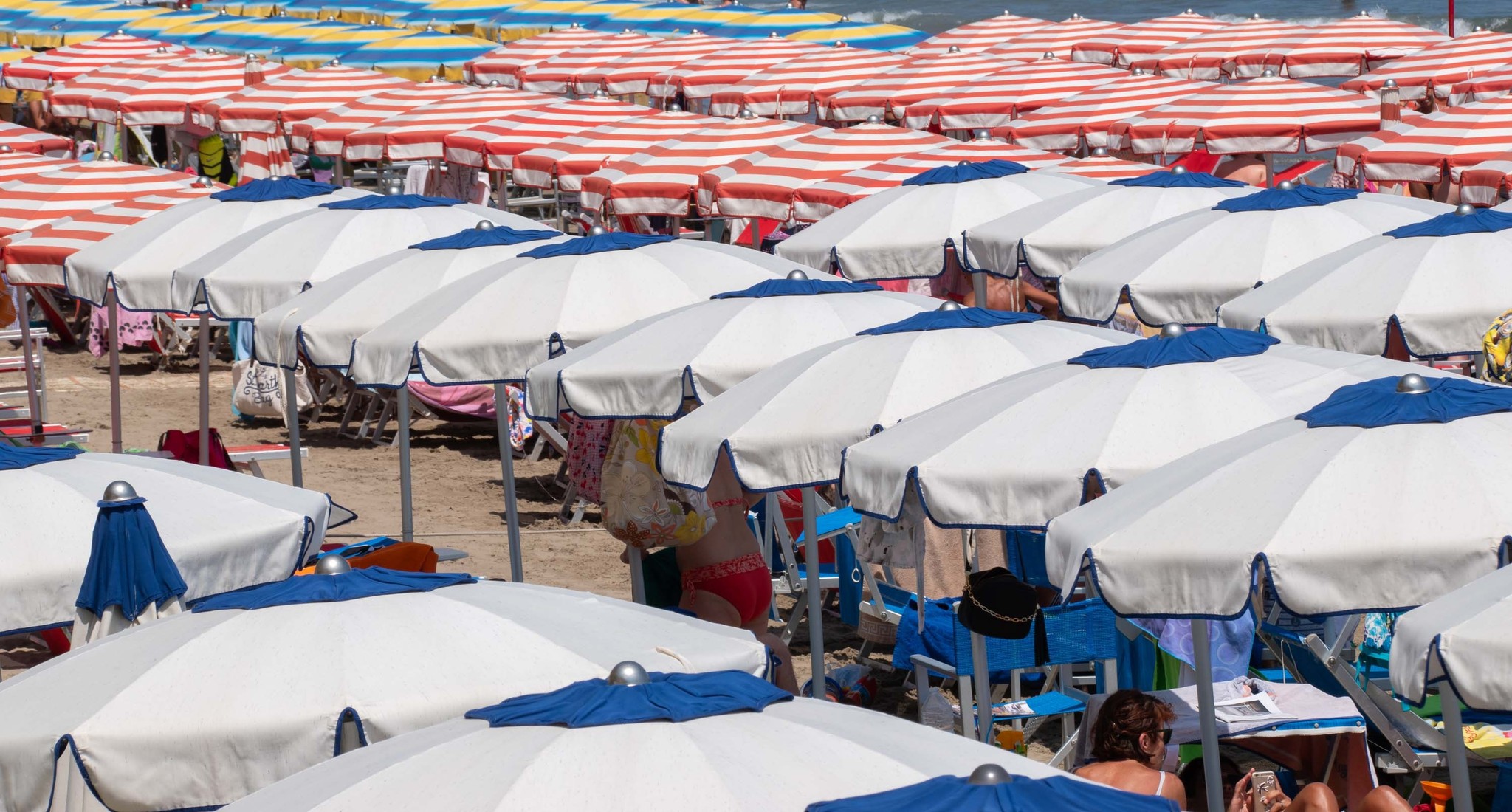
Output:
[[789, 0, 808, 11], [954, 257, 1058, 316], [227, 149, 238, 164], [677, 0, 704, 5], [657, 370, 801, 697], [1407, 82, 1450, 203], [718, 0, 732, 7], [1223, 768, 1415, 812], [654, 93, 696, 113], [1069, 688, 1186, 809], [0, 275, 16, 329], [1212, 152, 1273, 186], [851, 110, 900, 127], [1445, 354, 1470, 363], [29, 100, 78, 137]]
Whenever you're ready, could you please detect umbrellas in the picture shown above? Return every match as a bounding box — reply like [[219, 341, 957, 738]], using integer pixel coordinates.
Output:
[[0, 0, 1512, 812]]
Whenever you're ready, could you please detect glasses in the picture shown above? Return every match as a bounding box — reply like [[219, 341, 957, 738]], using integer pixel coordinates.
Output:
[[1157, 728, 1173, 744]]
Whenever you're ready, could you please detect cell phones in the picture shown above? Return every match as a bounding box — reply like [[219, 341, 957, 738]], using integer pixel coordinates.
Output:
[[1251, 770, 1278, 812]]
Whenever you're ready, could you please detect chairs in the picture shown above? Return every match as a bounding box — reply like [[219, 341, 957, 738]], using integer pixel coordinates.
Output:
[[153, 99, 1512, 812]]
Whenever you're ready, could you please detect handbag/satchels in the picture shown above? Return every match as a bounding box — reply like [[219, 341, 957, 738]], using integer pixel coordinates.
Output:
[[567, 411, 717, 545], [157, 428, 238, 471], [957, 567, 1050, 667], [232, 358, 314, 416]]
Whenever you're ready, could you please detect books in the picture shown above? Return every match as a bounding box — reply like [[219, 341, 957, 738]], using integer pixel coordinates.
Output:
[[1196, 692, 1299, 723]]
